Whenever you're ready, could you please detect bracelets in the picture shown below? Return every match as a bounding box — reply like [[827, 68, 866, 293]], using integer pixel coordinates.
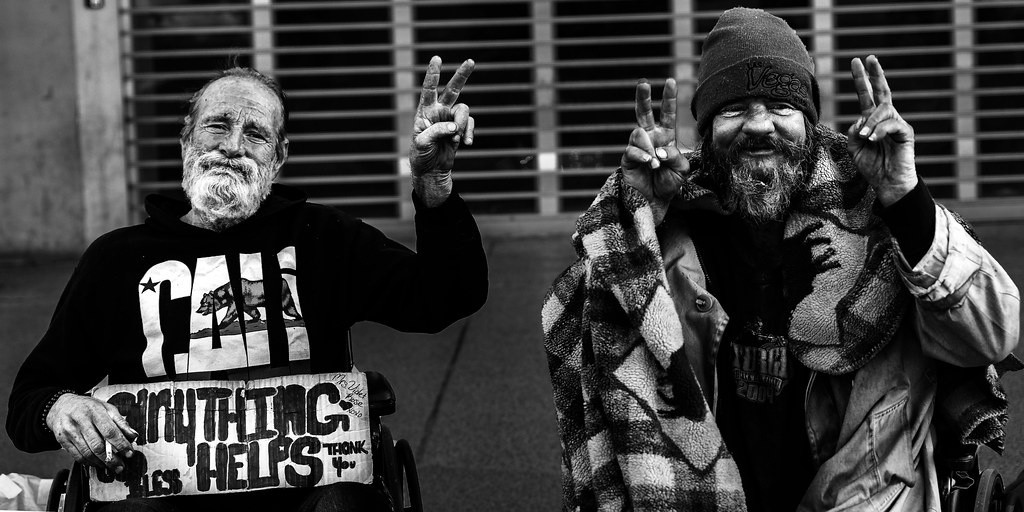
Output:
[[43, 389, 76, 433]]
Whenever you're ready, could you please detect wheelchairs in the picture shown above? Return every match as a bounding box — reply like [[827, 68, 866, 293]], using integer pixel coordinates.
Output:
[[934, 451, 1005, 512], [46, 371, 425, 512]]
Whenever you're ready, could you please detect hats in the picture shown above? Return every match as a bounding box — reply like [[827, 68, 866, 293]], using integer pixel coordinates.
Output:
[[690, 6, 821, 138]]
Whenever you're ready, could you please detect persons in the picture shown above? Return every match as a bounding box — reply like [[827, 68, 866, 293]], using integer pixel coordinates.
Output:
[[541, 7, 1021, 512], [5, 55, 490, 512]]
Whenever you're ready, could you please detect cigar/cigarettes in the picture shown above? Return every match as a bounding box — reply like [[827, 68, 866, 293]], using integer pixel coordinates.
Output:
[[104, 438, 112, 463]]
